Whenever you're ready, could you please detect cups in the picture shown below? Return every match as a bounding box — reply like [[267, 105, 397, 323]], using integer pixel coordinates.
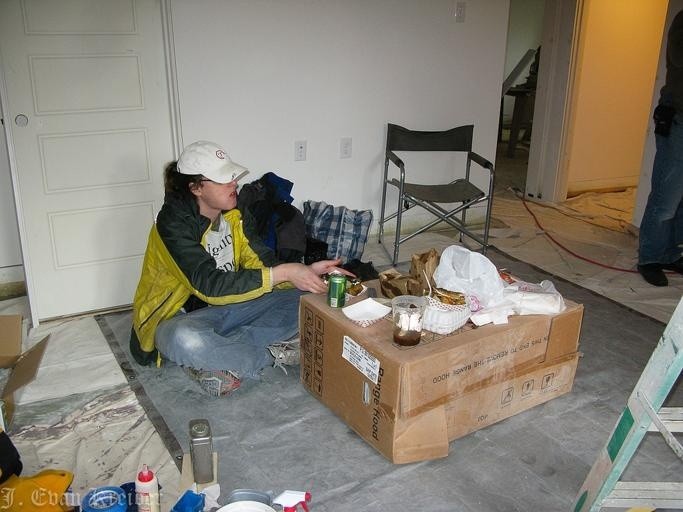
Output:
[[391, 295, 426, 347]]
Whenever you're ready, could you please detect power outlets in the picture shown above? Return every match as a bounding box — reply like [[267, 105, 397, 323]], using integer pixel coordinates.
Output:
[[454, 2, 467, 24]]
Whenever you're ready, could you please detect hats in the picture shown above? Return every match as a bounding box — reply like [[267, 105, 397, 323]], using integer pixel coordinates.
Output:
[[176, 141, 248, 184]]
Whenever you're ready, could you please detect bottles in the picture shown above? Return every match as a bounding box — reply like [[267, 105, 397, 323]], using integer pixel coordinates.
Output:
[[136, 464, 159, 512], [326, 274, 347, 308]]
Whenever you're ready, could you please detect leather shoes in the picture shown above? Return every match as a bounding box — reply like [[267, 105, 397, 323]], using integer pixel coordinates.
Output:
[[637, 262, 668, 287], [663, 258, 683, 275]]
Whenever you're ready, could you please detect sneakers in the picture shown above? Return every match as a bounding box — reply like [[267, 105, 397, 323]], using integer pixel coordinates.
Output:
[[181, 365, 241, 396], [267, 341, 300, 376]]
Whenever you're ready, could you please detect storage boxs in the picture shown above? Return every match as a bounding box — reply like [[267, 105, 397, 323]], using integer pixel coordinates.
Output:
[[2, 314, 51, 434], [297, 255, 585, 464]]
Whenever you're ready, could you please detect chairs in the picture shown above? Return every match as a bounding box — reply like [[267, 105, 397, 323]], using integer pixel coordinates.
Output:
[[377, 122, 498, 267]]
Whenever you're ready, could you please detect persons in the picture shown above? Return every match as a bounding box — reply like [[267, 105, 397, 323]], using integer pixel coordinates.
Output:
[[636, 10, 683, 287], [129, 141, 357, 396]]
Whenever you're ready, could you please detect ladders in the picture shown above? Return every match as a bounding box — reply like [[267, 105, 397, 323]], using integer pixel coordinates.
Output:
[[570, 295, 683, 511]]
